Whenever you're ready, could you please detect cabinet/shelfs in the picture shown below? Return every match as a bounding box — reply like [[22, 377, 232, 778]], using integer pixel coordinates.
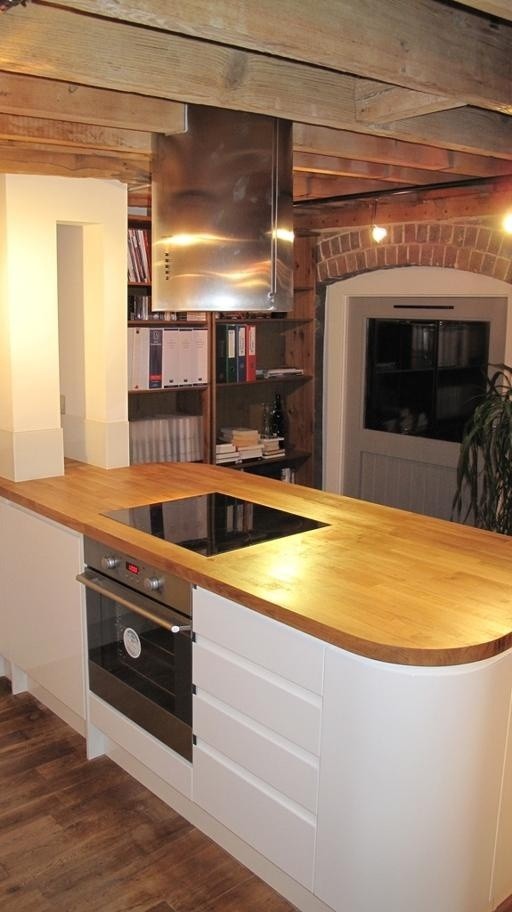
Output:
[[126, 212, 321, 490], [366, 318, 488, 444]]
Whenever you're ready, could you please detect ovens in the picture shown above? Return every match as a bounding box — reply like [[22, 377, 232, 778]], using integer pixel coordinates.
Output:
[[76, 534, 193, 765]]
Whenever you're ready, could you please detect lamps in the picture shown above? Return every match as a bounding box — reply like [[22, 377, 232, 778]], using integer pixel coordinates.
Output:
[[368, 197, 389, 243]]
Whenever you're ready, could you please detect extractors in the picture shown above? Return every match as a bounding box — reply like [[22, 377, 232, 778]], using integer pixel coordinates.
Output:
[[151, 104, 294, 313]]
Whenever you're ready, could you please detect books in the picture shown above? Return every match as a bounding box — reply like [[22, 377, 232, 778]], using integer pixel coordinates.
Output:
[[216, 323, 302, 466], [128, 227, 211, 461], [411, 325, 468, 419], [115, 493, 262, 555]]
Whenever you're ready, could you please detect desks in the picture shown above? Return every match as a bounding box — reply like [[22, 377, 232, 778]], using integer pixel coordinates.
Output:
[[0, 457, 511, 911]]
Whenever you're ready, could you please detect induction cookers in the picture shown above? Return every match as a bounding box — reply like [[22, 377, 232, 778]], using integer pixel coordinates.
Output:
[[98, 492, 331, 558]]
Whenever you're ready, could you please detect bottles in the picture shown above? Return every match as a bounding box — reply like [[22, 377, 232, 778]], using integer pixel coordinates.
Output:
[[259, 403, 274, 440], [272, 394, 289, 449]]
